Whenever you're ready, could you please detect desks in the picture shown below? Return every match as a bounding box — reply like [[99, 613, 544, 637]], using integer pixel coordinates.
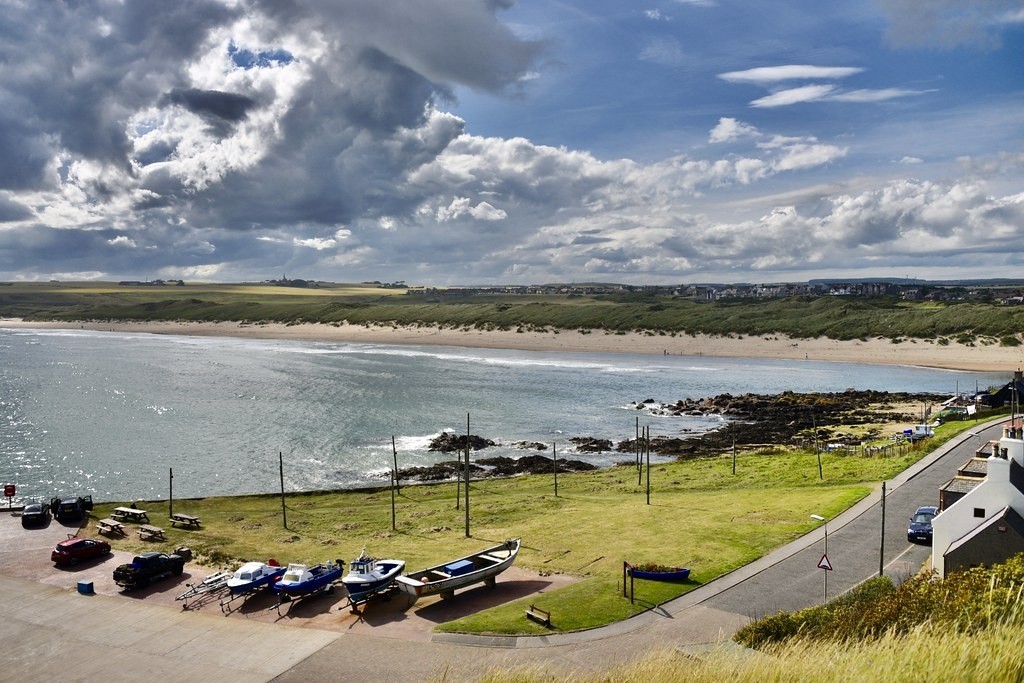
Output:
[[114, 507, 146, 526], [174, 513, 198, 529], [140, 524, 162, 541], [99, 518, 124, 537]]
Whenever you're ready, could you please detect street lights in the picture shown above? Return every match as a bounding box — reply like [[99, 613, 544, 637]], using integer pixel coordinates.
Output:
[[811, 514, 828, 605], [1008, 387, 1017, 425]]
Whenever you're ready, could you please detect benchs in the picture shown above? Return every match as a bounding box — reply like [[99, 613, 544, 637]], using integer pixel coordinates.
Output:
[[525, 604, 551, 628], [478, 554, 503, 565], [96, 513, 202, 536], [431, 569, 452, 580]]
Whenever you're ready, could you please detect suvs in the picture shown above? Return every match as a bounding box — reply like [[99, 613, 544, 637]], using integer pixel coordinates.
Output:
[[50, 495, 93, 521], [907, 505, 943, 543]]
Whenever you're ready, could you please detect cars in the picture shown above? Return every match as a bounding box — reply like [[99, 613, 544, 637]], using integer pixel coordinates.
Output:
[[22, 504, 49, 525], [974, 394, 994, 405], [51, 535, 112, 566]]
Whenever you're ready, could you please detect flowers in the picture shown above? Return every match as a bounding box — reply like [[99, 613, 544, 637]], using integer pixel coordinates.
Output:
[[632, 563, 681, 572]]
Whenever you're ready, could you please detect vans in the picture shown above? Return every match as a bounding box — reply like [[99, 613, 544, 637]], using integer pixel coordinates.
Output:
[[903, 429, 913, 436]]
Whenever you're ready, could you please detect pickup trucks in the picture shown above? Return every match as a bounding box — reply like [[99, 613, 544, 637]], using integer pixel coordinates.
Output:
[[112, 552, 185, 589]]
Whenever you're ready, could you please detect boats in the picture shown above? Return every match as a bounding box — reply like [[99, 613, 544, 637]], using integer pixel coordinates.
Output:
[[627, 562, 691, 580], [226, 559, 287, 592], [393, 538, 520, 600], [907, 425, 934, 443], [272, 559, 345, 596], [341, 549, 406, 600]]
